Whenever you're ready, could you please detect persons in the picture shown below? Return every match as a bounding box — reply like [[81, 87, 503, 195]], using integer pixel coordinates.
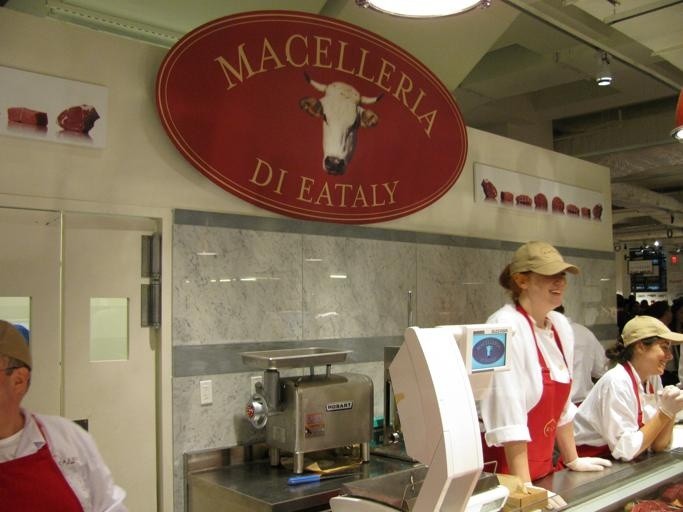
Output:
[[555, 303, 611, 407], [472, 241, 616, 510], [0, 320, 129, 512], [555, 315, 683, 460], [614, 293, 683, 388]]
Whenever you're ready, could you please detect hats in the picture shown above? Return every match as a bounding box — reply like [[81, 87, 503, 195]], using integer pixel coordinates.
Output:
[[509, 240, 579, 278], [621, 315, 683, 349], [0, 319, 33, 371]]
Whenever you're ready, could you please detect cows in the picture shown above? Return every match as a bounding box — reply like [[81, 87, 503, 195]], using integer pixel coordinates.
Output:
[[298, 69, 384, 176]]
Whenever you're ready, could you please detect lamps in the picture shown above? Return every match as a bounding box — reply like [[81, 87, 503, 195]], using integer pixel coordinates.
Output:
[[593, 0, 622, 88]]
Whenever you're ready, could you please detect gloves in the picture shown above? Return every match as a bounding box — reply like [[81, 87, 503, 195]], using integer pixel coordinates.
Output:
[[524, 482, 568, 511], [657, 385, 683, 421], [566, 457, 612, 472]]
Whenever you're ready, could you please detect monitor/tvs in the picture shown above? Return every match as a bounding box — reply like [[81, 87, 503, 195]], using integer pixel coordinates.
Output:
[[629, 256, 667, 294]]
